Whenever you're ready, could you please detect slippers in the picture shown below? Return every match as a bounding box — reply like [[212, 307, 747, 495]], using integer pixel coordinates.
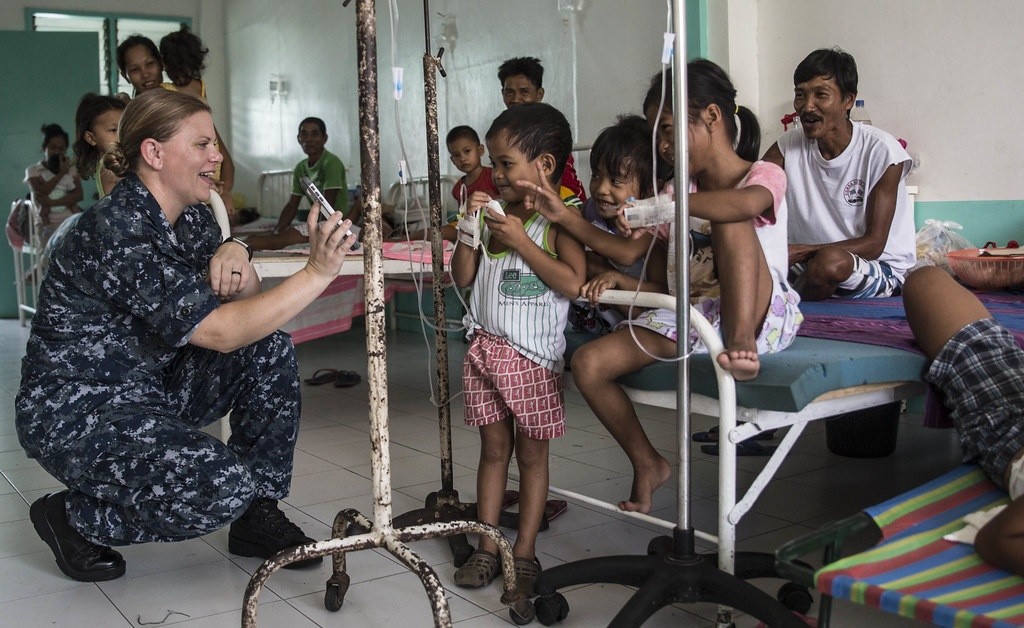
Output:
[[502, 489, 519, 511], [334, 370, 362, 387], [701, 441, 789, 456], [304, 368, 338, 385], [544, 499, 568, 522], [692, 421, 773, 442]]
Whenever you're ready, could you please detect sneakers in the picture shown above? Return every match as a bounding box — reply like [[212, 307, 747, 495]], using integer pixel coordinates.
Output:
[[30, 489, 126, 582], [227, 499, 323, 569]]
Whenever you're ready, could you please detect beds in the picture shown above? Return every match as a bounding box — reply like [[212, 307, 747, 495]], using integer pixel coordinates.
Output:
[[10, 169, 1024, 627]]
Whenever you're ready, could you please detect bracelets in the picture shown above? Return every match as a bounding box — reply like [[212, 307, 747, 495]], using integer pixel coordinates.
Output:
[[222, 236, 253, 263]]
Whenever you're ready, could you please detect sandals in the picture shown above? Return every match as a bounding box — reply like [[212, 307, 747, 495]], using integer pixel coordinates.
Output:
[[454, 549, 503, 589], [512, 555, 543, 599]]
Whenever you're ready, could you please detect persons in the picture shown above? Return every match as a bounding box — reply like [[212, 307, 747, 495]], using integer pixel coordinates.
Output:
[[115, 23, 236, 216], [403, 126, 501, 244], [227, 206, 259, 227], [16, 88, 356, 582], [516, 58, 803, 513], [451, 103, 586, 595], [760, 49, 916, 300], [73, 92, 126, 200], [24, 121, 84, 252], [246, 118, 349, 251], [902, 266, 1024, 576], [498, 57, 587, 206]]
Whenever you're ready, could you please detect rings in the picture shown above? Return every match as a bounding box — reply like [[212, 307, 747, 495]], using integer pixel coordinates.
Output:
[[232, 271, 241, 275]]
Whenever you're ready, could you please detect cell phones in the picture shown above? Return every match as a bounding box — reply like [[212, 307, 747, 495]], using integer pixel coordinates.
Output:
[[300, 177, 360, 250]]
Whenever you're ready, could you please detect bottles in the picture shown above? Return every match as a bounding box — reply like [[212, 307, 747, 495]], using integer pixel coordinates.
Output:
[[434, 15, 447, 51], [269, 77, 278, 103], [558, 0, 575, 33]]
